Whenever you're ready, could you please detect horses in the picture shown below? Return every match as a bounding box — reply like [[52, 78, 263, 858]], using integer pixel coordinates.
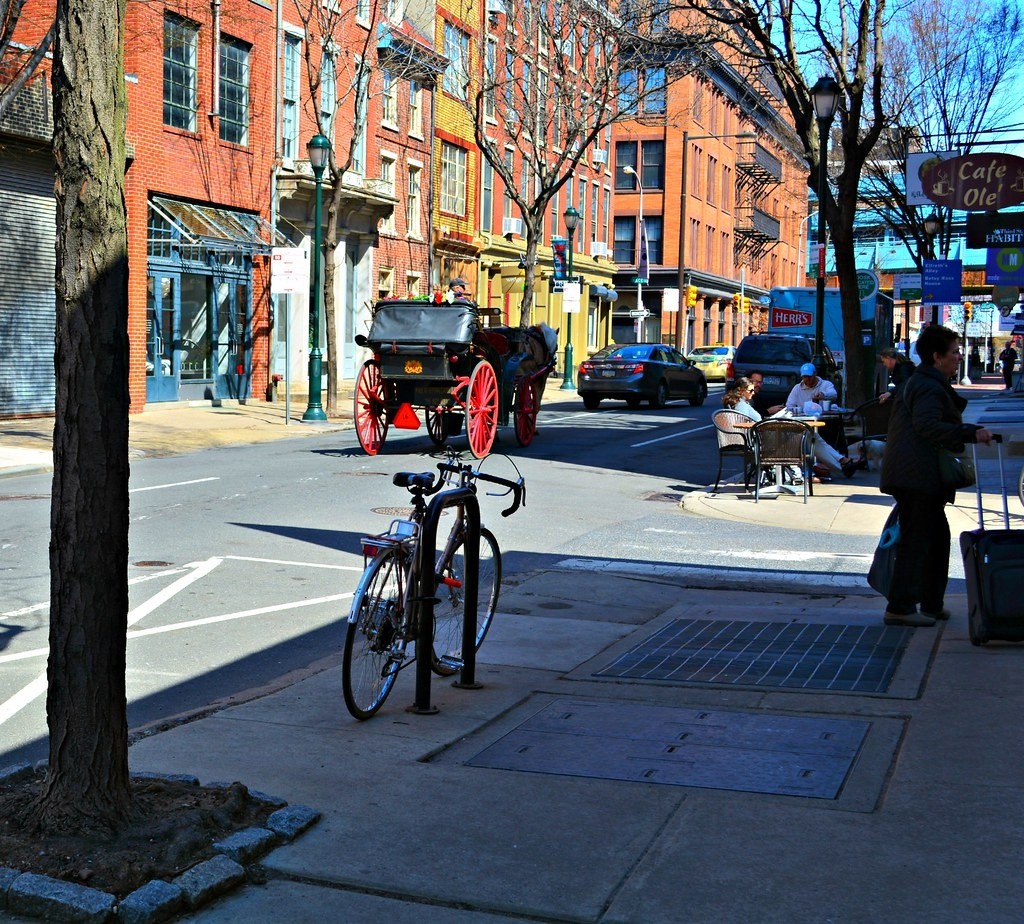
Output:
[[470, 321, 560, 442]]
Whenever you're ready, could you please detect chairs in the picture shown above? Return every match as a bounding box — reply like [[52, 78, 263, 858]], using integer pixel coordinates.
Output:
[[755, 418, 816, 506], [851, 396, 892, 472], [712, 409, 757, 493]]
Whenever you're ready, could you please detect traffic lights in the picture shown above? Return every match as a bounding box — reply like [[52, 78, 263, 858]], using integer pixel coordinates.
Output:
[[732, 294, 740, 313], [743, 297, 750, 313], [963, 302, 972, 320], [686, 285, 697, 307]]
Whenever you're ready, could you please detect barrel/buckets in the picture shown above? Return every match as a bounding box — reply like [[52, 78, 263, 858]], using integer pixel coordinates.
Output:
[[440, 413, 464, 435]]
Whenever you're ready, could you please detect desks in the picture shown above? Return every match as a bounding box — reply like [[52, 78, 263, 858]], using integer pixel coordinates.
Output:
[[788, 407, 856, 460], [732, 419, 825, 496]]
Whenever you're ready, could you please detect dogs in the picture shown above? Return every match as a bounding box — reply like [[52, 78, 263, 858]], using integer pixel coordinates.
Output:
[[856, 440, 886, 470]]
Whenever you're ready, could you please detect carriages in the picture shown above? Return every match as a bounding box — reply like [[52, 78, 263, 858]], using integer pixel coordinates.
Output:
[[352, 302, 560, 460]]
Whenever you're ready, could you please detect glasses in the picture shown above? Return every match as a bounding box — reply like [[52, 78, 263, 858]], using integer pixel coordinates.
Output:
[[752, 380, 765, 386], [749, 389, 754, 393]]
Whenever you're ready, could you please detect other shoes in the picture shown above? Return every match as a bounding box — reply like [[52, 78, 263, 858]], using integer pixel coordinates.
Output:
[[921, 605, 951, 620], [812, 475, 822, 483], [884, 611, 935, 627]]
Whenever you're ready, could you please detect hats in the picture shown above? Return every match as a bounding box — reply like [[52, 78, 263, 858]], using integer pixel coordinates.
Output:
[[449, 278, 469, 286], [800, 363, 816, 376]]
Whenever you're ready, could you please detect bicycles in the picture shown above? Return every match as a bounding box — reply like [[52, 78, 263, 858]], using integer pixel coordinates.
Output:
[[342, 445, 527, 723]]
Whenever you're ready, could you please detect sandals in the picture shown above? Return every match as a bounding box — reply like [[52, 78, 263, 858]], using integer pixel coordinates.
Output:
[[840, 458, 866, 470], [843, 464, 858, 478]]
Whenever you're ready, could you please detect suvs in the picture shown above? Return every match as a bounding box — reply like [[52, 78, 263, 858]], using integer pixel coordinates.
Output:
[[724, 331, 845, 410]]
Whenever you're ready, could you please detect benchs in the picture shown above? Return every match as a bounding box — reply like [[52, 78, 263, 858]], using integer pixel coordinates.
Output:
[[360, 309, 484, 379]]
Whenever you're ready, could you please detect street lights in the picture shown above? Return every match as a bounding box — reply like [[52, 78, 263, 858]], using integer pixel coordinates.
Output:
[[558, 207, 581, 389], [923, 209, 940, 327], [673, 131, 758, 354], [622, 166, 643, 357], [807, 73, 841, 381], [299, 135, 332, 424], [796, 211, 818, 287]]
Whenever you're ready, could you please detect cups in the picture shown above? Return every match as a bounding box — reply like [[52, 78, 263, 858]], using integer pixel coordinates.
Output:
[[812, 395, 819, 404], [786, 403, 801, 413], [822, 401, 831, 411]]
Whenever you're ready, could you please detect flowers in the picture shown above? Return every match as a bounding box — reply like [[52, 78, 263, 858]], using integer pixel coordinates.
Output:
[[373, 291, 457, 304]]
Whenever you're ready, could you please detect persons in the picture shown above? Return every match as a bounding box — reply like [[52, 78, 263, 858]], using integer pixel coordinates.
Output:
[[746, 368, 820, 481], [999, 342, 1017, 390], [879, 347, 917, 403], [723, 374, 867, 478], [879, 324, 992, 626], [448, 279, 469, 291], [786, 363, 837, 477]]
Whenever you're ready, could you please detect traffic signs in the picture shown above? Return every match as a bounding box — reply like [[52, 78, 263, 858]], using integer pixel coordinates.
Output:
[[629, 308, 651, 318]]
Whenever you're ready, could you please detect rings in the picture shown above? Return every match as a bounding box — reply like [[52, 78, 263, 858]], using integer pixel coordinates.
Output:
[[883, 398, 885, 400]]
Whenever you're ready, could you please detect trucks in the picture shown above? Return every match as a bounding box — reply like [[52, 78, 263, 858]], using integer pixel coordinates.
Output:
[[766, 286, 903, 409]]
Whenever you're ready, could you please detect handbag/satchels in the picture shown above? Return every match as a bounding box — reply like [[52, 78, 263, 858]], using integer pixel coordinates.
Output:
[[867, 506, 898, 601], [934, 447, 978, 489]]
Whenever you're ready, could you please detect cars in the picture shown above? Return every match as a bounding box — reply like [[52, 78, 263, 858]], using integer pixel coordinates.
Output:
[[576, 343, 708, 410], [679, 343, 737, 382]]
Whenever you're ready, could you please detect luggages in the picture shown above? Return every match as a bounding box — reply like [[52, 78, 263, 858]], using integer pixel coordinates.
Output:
[[959, 432, 1023, 646]]
